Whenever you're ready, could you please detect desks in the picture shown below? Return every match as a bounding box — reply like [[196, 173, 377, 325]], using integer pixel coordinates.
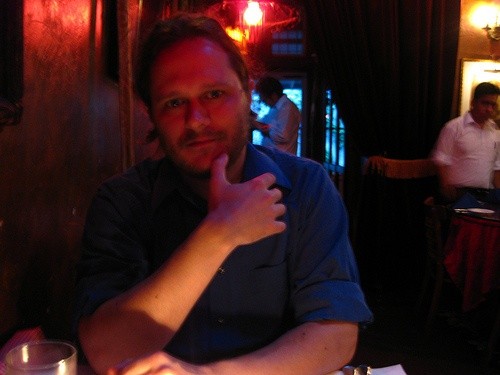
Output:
[[437, 207, 500, 311]]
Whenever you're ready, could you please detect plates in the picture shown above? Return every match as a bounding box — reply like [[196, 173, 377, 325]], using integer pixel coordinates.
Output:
[[467, 207, 495, 214]]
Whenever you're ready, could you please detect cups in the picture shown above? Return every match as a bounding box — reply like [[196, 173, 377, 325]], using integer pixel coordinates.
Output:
[[3, 341, 77, 375]]
